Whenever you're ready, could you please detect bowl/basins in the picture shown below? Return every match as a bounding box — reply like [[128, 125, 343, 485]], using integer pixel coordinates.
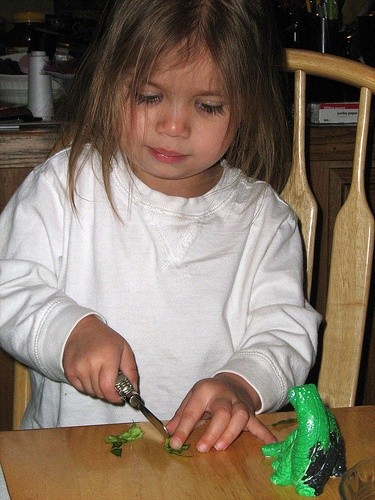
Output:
[[0, 53, 65, 103]]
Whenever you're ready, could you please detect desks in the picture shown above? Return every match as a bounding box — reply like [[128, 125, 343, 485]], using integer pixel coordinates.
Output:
[[0, 405, 375, 500]]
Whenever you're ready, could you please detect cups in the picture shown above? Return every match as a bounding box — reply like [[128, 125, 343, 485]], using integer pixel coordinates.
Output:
[[314, 19, 345, 103]]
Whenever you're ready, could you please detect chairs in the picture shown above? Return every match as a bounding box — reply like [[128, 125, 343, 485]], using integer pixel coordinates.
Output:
[[12, 46, 375, 431]]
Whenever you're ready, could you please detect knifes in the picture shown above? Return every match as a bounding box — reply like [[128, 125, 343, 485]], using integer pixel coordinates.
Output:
[[115, 371, 169, 439]]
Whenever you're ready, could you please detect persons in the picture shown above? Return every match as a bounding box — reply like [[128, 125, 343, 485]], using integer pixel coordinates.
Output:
[[0, 0, 324, 454]]
